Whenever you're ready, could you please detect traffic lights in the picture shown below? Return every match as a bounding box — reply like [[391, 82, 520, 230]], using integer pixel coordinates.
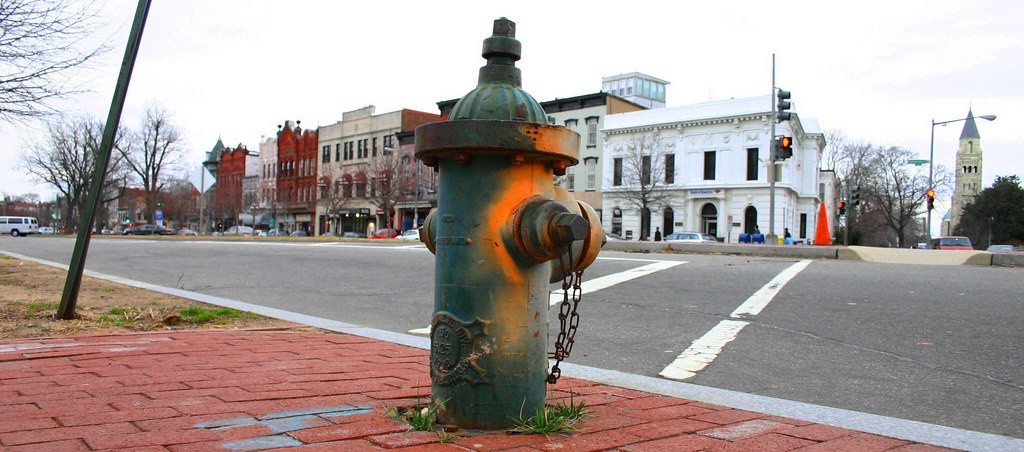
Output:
[[927, 190, 935, 210], [837, 201, 846, 216], [849, 185, 860, 207], [776, 89, 792, 122], [778, 137, 793, 159], [126, 219, 131, 224]]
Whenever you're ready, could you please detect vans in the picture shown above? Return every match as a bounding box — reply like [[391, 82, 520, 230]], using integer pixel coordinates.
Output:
[[0, 215, 39, 237], [930, 235, 974, 252]]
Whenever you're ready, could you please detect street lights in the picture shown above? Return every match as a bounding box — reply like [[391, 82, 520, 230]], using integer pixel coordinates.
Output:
[[926, 115, 996, 251]]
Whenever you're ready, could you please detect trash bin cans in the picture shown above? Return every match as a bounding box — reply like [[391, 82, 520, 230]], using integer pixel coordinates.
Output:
[[739, 233, 751, 244], [753, 234, 765, 243]]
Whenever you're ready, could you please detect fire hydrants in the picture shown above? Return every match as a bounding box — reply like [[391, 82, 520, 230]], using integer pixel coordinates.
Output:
[[414, 14, 608, 428]]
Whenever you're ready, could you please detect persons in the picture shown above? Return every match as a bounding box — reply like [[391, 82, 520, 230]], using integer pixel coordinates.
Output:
[[784, 228, 791, 237], [754, 225, 760, 234], [655, 227, 661, 241]]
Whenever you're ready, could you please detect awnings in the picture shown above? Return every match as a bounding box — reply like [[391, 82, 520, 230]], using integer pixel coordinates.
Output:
[[328, 208, 370, 214]]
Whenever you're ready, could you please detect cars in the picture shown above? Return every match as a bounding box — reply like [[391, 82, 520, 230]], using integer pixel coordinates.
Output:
[[396, 230, 419, 241], [916, 243, 926, 249], [987, 245, 1015, 251], [211, 226, 400, 239], [39, 227, 53, 234], [123, 224, 175, 236], [93, 227, 123, 235], [664, 233, 718, 244], [178, 229, 198, 237]]
